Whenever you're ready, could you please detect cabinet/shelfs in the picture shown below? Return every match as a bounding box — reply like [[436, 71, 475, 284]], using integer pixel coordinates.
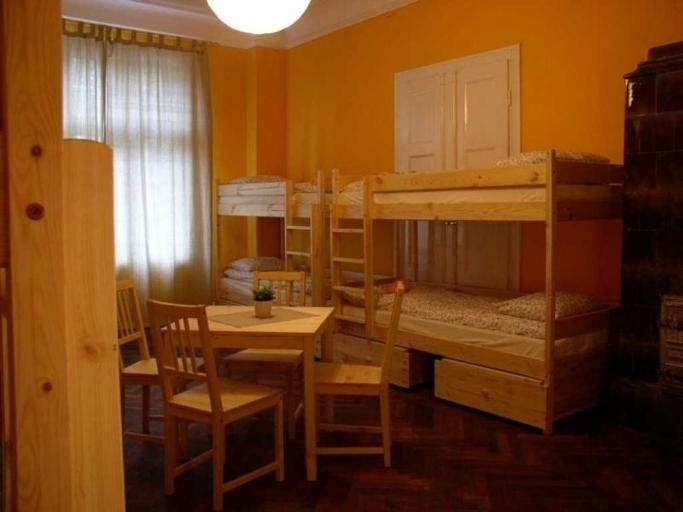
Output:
[[606, 40, 682, 449]]
